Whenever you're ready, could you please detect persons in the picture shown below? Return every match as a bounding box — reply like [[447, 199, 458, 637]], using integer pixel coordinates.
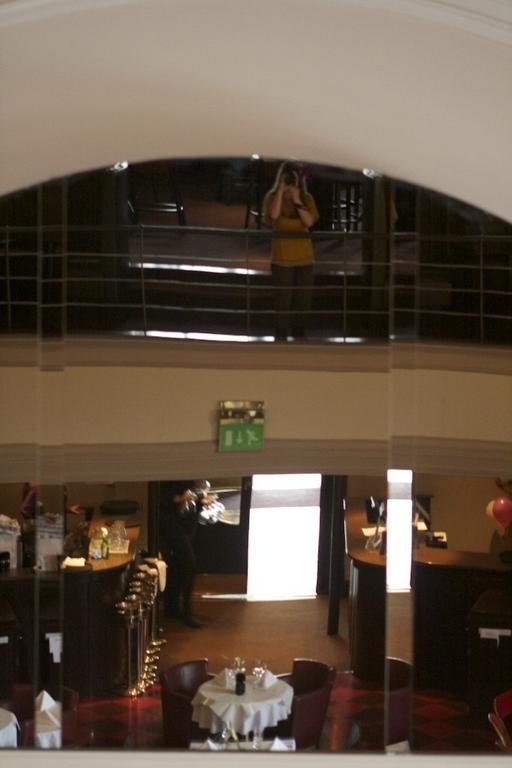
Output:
[[352, 183, 398, 309], [263, 161, 322, 342], [20, 483, 82, 566], [159, 480, 214, 628]]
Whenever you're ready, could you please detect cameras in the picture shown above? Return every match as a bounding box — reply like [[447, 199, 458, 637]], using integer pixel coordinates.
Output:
[[285, 174, 296, 186]]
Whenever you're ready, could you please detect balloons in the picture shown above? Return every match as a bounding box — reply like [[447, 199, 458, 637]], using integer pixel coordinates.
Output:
[[491, 496, 512, 529], [485, 499, 505, 537]]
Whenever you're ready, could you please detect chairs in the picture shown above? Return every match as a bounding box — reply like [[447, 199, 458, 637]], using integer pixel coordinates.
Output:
[[279, 656, 337, 750], [158, 656, 216, 750], [485, 684, 512, 755]]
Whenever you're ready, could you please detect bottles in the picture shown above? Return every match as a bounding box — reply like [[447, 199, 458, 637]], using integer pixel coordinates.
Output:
[[101, 527, 109, 559]]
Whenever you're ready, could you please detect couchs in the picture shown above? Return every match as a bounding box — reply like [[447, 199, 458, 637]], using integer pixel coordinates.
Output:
[[127, 200, 272, 308]]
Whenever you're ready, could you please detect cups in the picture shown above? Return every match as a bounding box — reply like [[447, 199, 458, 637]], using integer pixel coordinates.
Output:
[[252, 726, 264, 750]]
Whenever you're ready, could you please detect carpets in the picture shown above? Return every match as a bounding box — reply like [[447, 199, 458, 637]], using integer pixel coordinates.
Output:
[[1, 673, 512, 754]]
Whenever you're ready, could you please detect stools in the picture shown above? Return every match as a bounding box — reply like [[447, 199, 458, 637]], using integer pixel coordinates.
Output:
[[116, 555, 165, 712]]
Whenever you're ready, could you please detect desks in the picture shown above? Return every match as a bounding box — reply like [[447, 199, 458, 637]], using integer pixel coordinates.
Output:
[[1, 704, 19, 748], [190, 676, 293, 752]]
